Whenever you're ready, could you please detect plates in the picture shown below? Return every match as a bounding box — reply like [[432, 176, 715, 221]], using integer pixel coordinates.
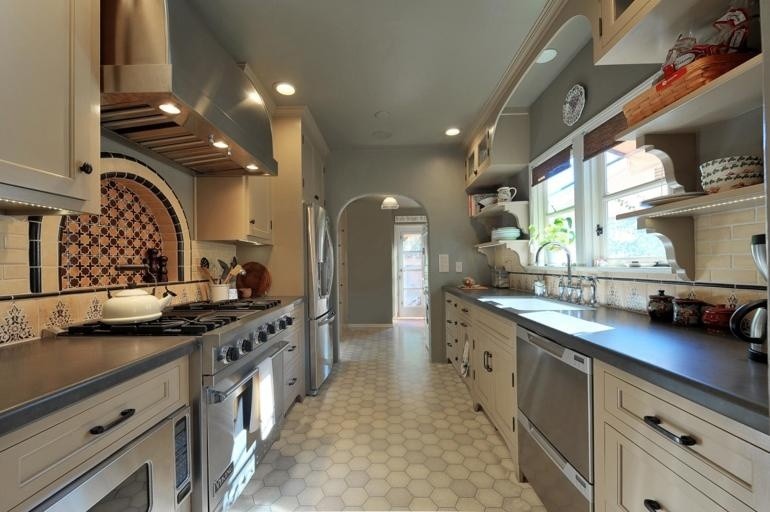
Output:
[[561, 83, 585, 127], [640, 191, 709, 207]]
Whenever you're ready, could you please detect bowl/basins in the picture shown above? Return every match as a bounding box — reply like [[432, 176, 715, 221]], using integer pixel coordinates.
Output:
[[491, 226, 522, 241], [698, 154, 763, 198], [479, 196, 497, 207]]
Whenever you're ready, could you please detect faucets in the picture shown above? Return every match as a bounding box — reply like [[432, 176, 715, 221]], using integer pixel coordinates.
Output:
[[535, 241, 574, 303], [576, 273, 599, 308]]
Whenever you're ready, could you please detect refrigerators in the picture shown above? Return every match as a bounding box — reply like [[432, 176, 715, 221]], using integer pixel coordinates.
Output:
[[303, 202, 337, 395]]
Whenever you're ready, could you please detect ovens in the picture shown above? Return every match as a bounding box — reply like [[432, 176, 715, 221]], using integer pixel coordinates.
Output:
[[203, 333, 292, 511]]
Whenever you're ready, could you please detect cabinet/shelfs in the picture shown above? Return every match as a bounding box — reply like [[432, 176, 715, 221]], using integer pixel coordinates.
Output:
[[589, 0, 746, 66], [594, 357, 769, 512], [599, 1, 770, 285], [462, 111, 532, 268], [1, 0, 104, 218], [193, 174, 273, 247], [441, 286, 517, 480], [283, 315, 306, 415], [1, 354, 191, 511]]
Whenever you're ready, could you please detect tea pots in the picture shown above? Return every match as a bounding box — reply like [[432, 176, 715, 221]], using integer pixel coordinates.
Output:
[[96, 263, 177, 323]]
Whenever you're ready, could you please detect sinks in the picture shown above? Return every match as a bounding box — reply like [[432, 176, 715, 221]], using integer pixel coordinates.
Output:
[[478, 295, 598, 311]]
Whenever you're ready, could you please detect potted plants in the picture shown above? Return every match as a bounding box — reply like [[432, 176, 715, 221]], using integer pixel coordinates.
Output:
[[528, 215, 577, 269]]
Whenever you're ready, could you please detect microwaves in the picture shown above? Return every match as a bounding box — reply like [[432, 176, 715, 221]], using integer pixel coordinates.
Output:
[[30, 405, 194, 512]]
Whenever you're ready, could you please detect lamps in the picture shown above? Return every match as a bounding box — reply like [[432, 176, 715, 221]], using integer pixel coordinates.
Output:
[[381, 196, 400, 210]]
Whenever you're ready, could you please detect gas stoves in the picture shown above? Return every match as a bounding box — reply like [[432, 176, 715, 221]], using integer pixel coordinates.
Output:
[[57, 296, 282, 337]]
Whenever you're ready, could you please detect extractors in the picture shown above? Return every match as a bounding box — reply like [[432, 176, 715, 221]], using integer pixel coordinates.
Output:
[[101, 2, 283, 180]]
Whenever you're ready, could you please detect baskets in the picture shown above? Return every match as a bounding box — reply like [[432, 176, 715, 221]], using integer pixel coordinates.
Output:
[[621, 53, 751, 128]]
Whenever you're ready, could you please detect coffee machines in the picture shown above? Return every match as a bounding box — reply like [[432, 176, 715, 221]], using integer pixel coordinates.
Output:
[[729, 232, 769, 365]]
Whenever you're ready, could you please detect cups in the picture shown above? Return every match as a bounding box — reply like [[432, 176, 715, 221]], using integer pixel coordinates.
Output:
[[534, 281, 544, 297], [497, 185, 517, 203], [209, 284, 252, 301]]
